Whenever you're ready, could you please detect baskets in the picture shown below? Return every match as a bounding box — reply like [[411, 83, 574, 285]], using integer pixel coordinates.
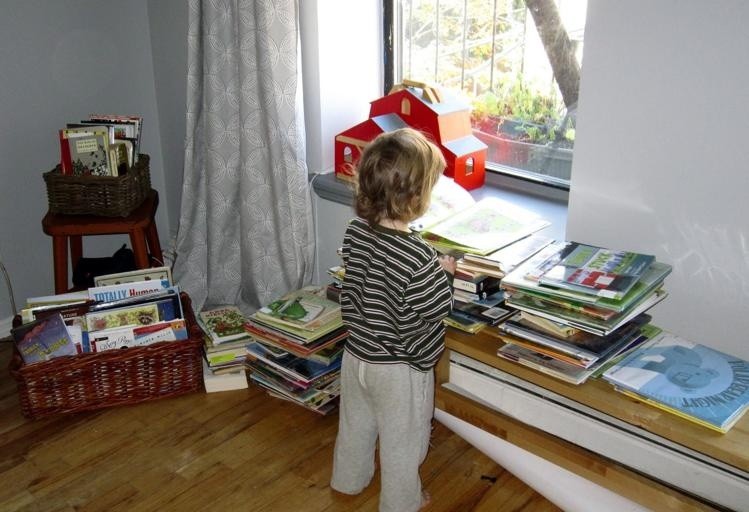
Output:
[[8, 291, 201, 417], [43, 154, 150, 218]]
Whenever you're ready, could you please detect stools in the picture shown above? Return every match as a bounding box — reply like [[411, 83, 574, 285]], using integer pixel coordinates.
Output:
[[41, 188, 165, 297]]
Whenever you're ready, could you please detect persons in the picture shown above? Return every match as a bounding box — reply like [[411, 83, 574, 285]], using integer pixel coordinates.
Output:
[[327, 128, 458, 512]]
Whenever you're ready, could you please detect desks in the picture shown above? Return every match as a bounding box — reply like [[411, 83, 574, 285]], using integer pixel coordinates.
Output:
[[327, 281, 749, 512]]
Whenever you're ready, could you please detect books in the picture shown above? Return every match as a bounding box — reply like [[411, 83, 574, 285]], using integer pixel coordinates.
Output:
[[409, 171, 748, 434], [413, 175, 673, 385], [11, 267, 188, 365], [604, 329, 748, 434], [59, 112, 143, 177], [194, 305, 252, 393], [247, 286, 343, 416]]
